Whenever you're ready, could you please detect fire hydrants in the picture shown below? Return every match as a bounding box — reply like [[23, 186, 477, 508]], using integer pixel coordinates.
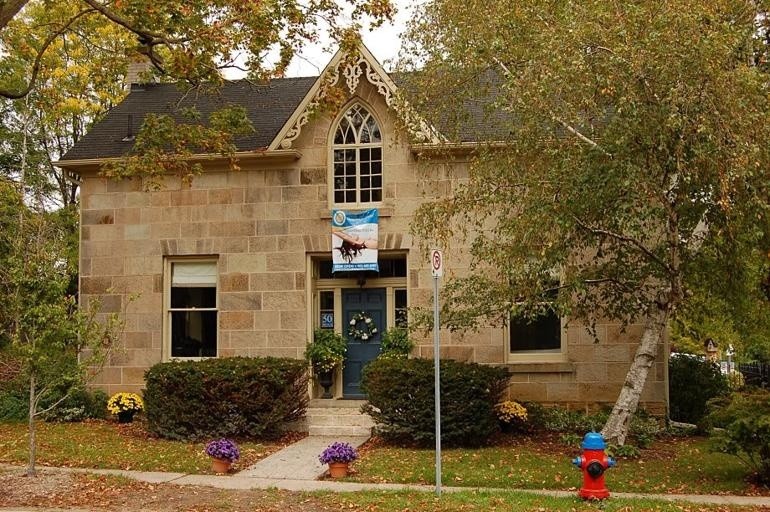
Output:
[[570, 433, 616, 501]]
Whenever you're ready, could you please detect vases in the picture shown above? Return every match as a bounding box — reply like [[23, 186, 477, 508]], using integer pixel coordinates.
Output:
[[320, 370, 334, 399], [329, 462, 349, 477], [502, 424, 513, 433], [117, 412, 133, 424], [212, 459, 231, 473]]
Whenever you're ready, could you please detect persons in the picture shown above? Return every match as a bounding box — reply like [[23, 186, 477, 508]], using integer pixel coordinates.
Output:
[[334, 228, 379, 255]]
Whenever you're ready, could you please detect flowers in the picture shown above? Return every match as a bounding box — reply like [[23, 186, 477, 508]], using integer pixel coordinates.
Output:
[[107, 392, 145, 415], [494, 399, 529, 424], [317, 442, 358, 465], [304, 328, 349, 376], [205, 437, 240, 463]]
